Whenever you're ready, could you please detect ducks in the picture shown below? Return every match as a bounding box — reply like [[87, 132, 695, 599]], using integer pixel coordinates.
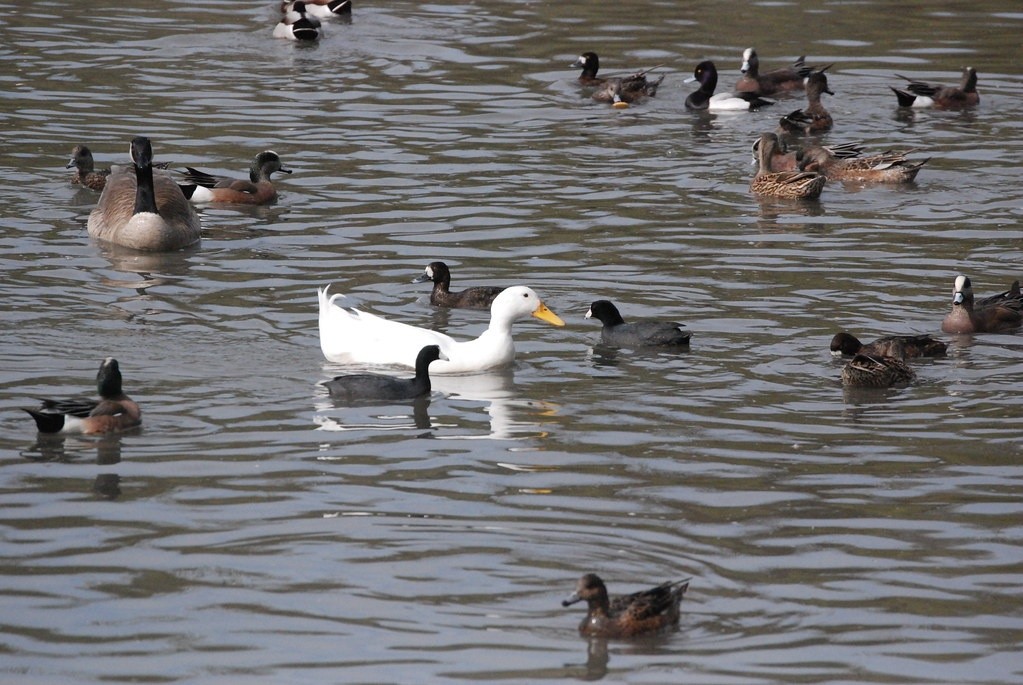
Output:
[[317, 283, 565, 372]]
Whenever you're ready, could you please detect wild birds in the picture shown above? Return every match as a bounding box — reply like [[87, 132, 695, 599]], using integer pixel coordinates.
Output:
[[890, 66, 980, 108], [569, 51, 665, 108], [410, 261, 506, 311], [684, 47, 932, 201], [585, 299, 691, 349], [561, 575, 696, 634], [831, 333, 952, 388], [66, 138, 292, 253], [272, 0, 351, 41], [319, 345, 450, 400], [19, 356, 140, 434], [942, 275, 1023, 333]]
